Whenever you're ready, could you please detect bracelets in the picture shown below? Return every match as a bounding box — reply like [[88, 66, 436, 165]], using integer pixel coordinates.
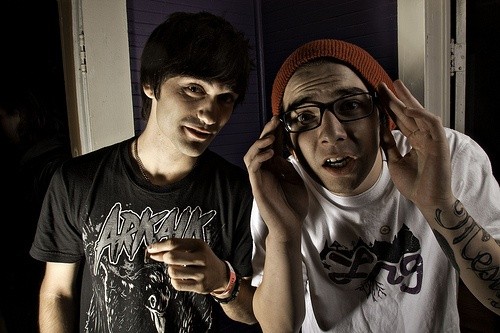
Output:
[[210, 260, 242, 304]]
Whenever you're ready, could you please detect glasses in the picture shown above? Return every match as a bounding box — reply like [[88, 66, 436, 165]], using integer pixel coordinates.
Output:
[[278, 90, 378, 132]]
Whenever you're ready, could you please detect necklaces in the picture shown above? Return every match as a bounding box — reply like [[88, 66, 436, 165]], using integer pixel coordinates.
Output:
[[134, 137, 153, 184]]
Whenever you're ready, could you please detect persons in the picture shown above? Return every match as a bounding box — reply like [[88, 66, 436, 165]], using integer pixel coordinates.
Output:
[[0, 88, 67, 333], [29, 11, 262, 333], [243, 39, 500, 333]]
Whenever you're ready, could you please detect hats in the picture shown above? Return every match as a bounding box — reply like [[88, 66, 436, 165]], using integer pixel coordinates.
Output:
[[272, 38, 396, 156]]
